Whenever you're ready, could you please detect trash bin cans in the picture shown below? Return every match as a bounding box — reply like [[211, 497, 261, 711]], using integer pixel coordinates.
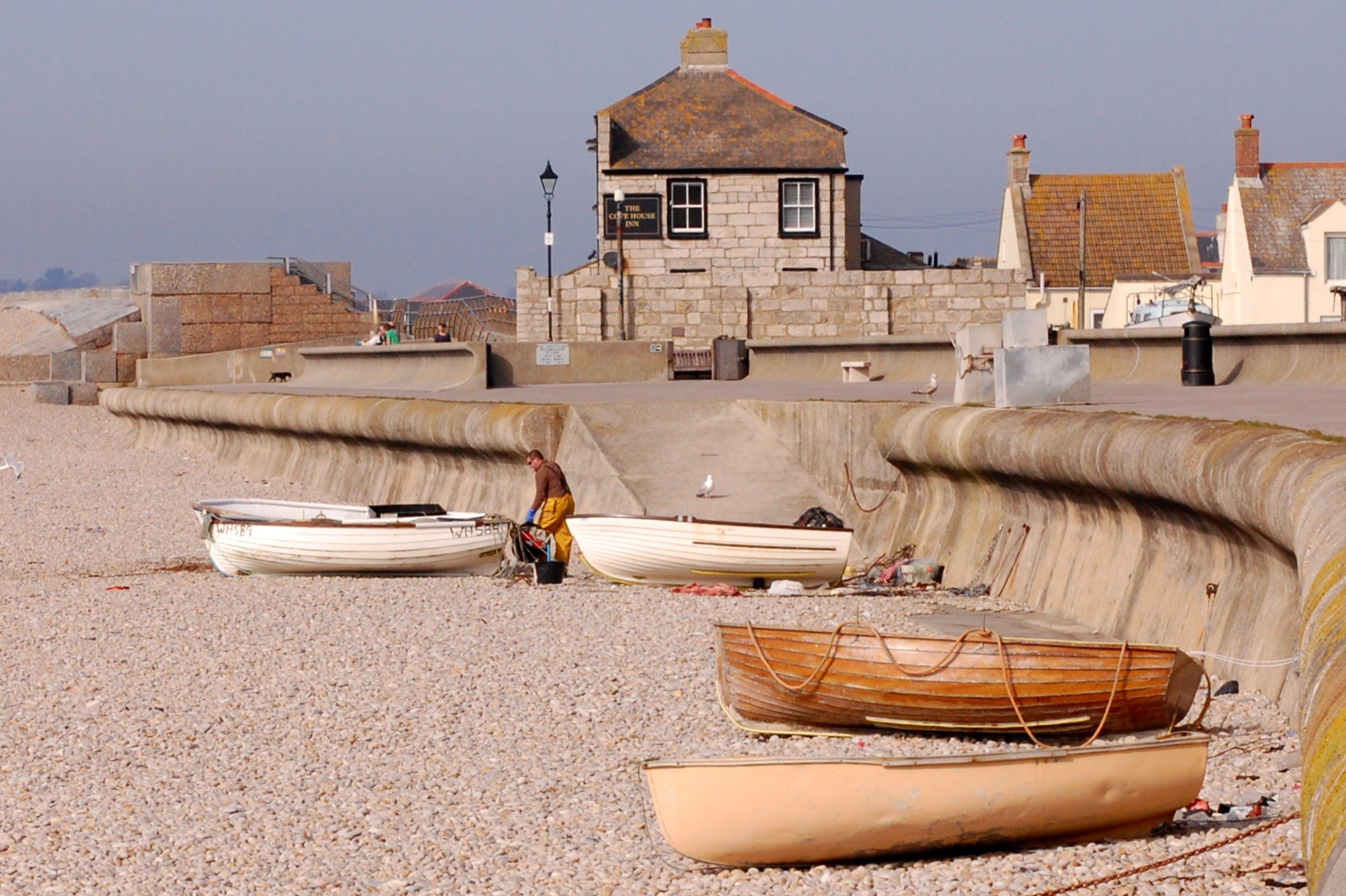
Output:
[[1180, 321, 1215, 385]]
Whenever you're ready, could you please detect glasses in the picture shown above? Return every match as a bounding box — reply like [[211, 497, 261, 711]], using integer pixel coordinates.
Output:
[[528, 458, 534, 465]]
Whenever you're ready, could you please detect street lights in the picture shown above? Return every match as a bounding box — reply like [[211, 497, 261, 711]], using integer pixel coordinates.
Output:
[[539, 160, 558, 342], [613, 190, 626, 343]]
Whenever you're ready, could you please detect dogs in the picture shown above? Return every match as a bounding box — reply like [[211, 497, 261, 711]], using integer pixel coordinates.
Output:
[[268, 371, 292, 383]]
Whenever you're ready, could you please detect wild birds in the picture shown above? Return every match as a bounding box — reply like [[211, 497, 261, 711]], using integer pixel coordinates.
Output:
[[910, 373, 938, 403], [695, 475, 715, 499]]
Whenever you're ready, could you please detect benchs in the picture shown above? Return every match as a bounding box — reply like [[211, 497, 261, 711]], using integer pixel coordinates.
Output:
[[840, 362, 871, 382]]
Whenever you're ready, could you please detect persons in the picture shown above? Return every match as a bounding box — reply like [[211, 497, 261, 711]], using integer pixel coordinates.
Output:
[[433, 324, 452, 344], [356, 321, 401, 348], [523, 449, 575, 580]]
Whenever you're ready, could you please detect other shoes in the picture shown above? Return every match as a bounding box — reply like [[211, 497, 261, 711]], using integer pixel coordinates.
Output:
[[562, 571, 568, 579]]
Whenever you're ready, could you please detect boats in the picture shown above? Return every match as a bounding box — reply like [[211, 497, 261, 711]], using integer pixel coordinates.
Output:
[[1126, 275, 1222, 328], [711, 617, 1204, 736], [565, 513, 854, 587], [192, 496, 510, 577], [643, 734, 1212, 869]]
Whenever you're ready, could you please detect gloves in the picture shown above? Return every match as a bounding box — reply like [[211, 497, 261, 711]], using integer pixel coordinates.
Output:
[[525, 507, 535, 523]]
